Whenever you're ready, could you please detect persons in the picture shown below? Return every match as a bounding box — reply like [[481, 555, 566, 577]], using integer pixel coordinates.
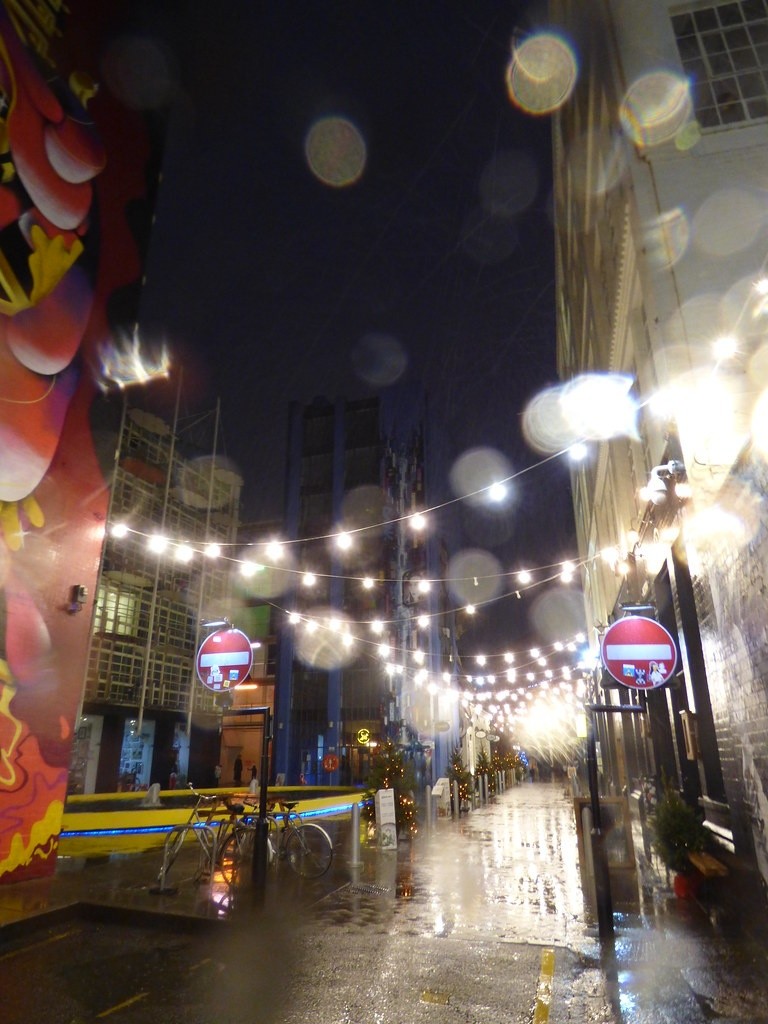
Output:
[[567, 762, 581, 796], [247, 764, 257, 784], [168, 768, 177, 790], [234, 753, 243, 786]]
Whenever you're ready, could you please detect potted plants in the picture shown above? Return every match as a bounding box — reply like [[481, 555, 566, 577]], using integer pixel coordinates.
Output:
[[649, 788, 713, 900]]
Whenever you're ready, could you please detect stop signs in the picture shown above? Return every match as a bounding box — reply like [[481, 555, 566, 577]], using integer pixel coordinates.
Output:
[[196, 629, 254, 695], [601, 615, 679, 692]]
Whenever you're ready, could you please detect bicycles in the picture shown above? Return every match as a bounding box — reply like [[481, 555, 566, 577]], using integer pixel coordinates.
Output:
[[156, 778, 335, 890]]
